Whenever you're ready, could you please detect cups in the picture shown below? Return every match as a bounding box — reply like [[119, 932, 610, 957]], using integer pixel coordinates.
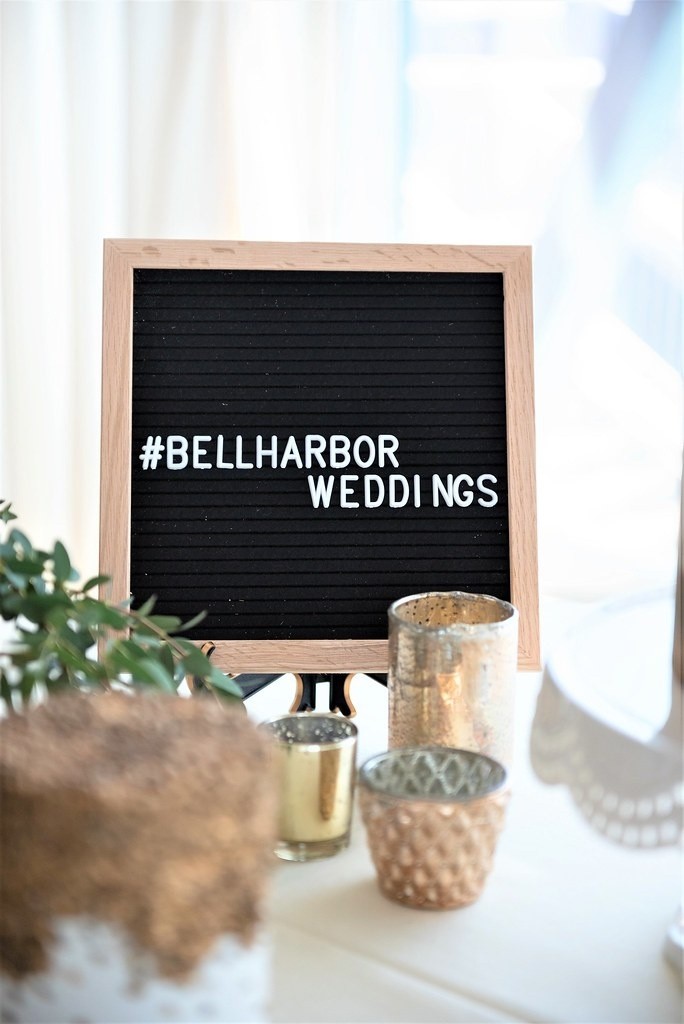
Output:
[[361, 749, 508, 909], [386, 591, 518, 757], [265, 717, 359, 866]]
